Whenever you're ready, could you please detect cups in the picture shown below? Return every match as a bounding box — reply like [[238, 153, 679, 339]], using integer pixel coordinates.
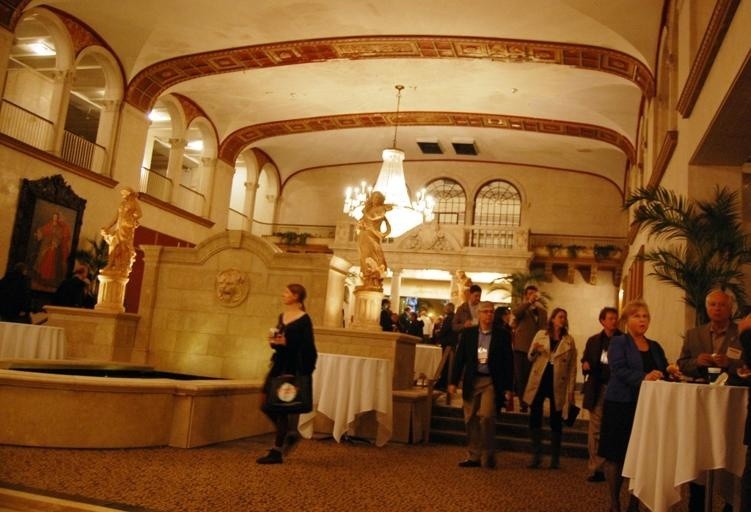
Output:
[[707, 366, 722, 386], [270, 328, 281, 338]]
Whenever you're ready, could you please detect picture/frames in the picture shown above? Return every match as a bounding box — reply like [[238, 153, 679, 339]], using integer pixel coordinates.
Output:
[[8, 173, 88, 303]]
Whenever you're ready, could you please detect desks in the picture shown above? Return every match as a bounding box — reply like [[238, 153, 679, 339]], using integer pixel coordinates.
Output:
[[619, 378, 750, 512], [415, 343, 445, 390], [296, 350, 396, 450]]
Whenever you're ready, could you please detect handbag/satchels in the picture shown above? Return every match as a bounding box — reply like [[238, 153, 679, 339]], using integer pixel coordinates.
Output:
[[562, 403, 580, 427], [269, 375, 304, 407]]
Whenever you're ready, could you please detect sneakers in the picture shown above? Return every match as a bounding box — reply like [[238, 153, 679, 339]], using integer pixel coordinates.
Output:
[[586, 471, 606, 482], [458, 460, 482, 467], [256, 448, 283, 464]]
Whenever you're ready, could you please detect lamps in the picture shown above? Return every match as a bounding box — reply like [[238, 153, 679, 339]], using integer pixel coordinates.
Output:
[[343, 84, 438, 242]]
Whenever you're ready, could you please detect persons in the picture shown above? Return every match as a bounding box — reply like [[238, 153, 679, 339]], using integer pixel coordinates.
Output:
[[596, 300, 682, 511], [33, 210, 71, 293], [256, 280, 318, 466], [1, 262, 51, 327], [355, 191, 395, 289], [380, 287, 547, 413], [677, 286, 750, 386], [55, 262, 99, 309], [99, 185, 146, 278], [448, 301, 514, 467], [582, 307, 623, 482], [523, 307, 577, 469]]
